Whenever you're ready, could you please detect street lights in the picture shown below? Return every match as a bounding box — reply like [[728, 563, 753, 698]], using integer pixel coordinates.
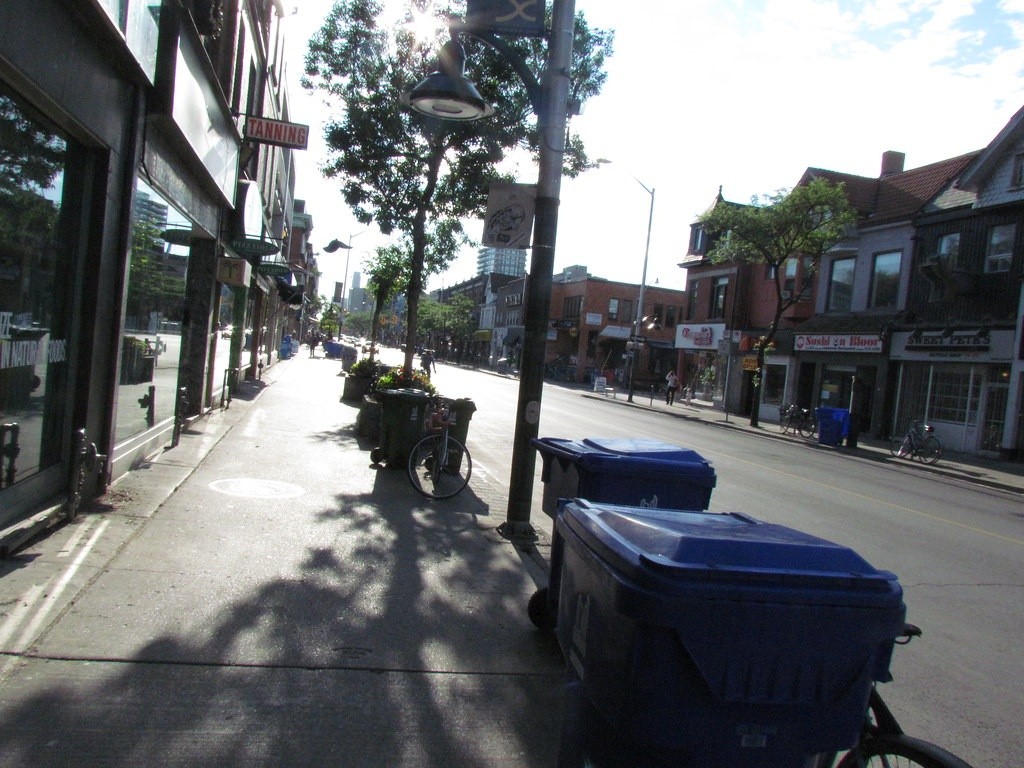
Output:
[[338, 232, 361, 342], [628, 177, 656, 401], [398, 0, 574, 542]]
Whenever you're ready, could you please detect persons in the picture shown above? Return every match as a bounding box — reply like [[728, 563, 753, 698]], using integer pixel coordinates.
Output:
[[665, 371, 678, 406], [686, 388, 693, 405], [308, 334, 317, 358], [422, 350, 436, 380]]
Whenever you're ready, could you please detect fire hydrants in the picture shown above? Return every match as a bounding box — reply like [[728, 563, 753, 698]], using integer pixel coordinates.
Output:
[[172, 387, 190, 447], [66, 428, 106, 520], [227, 367, 239, 408]]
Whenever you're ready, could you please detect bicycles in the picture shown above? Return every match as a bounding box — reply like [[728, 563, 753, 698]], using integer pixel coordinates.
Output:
[[780, 403, 817, 440], [407, 394, 472, 500], [812, 624, 972, 768], [889, 418, 942, 464]]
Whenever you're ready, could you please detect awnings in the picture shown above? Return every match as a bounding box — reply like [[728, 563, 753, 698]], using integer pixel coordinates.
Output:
[[474, 330, 492, 341], [503, 335, 519, 346]]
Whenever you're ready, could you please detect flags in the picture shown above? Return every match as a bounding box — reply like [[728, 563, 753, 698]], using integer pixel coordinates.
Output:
[[296, 308, 302, 322]]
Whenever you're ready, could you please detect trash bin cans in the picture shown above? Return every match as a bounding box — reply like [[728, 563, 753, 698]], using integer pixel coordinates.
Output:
[[342, 346, 358, 372], [424, 396, 477, 476], [555, 497, 907, 768], [371, 388, 432, 470], [814, 407, 850, 447], [0, 325, 50, 410], [528, 437, 717, 647]]
